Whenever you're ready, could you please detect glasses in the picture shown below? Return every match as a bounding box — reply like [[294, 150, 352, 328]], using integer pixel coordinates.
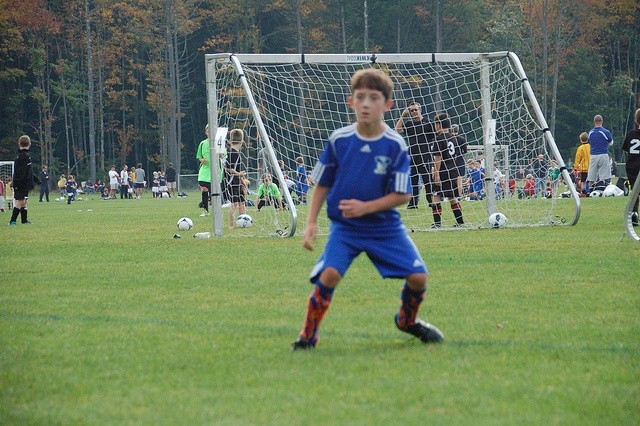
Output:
[[410, 109, 420, 112]]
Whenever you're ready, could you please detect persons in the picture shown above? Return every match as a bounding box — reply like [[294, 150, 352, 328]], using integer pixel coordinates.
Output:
[[224, 128, 247, 229], [573, 131, 591, 198], [196, 124, 228, 217], [130, 166, 137, 197], [57, 174, 110, 205], [158, 173, 172, 198], [5, 172, 14, 211], [120, 164, 130, 199], [39, 165, 50, 202], [127, 171, 134, 198], [532, 153, 548, 199], [144, 181, 148, 188], [430, 113, 465, 229], [621, 108, 640, 226], [545, 182, 552, 198], [584, 115, 614, 197], [463, 151, 516, 201], [394, 101, 438, 210], [292, 68, 443, 349], [520, 165, 524, 178], [255, 136, 315, 213], [449, 123, 468, 201], [135, 162, 145, 198], [157, 172, 162, 197], [547, 159, 560, 199], [517, 174, 537, 199], [565, 157, 574, 172], [108, 165, 120, 199], [0, 179, 5, 212], [165, 163, 176, 197], [9, 134, 33, 225], [152, 171, 160, 198]]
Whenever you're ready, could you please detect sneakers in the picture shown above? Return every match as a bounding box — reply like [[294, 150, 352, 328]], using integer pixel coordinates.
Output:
[[632, 207, 639, 226], [22, 221, 32, 224], [453, 223, 464, 227], [292, 335, 318, 351], [395, 312, 445, 343], [9, 221, 16, 225], [406, 204, 418, 209], [431, 224, 441, 229], [199, 209, 209, 217]]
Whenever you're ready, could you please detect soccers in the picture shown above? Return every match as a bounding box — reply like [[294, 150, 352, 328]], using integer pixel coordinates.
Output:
[[589, 190, 601, 198], [176, 217, 194, 232], [488, 213, 508, 230], [236, 213, 253, 229]]
